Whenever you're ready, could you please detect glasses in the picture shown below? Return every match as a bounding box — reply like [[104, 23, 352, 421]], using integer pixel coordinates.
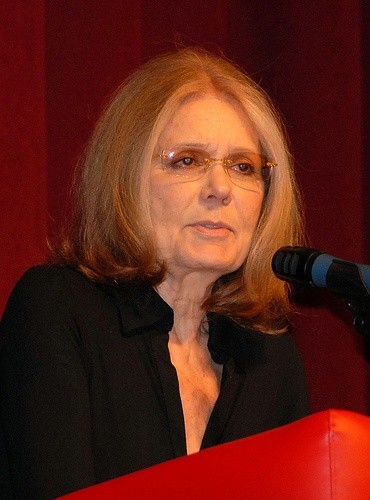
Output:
[[152, 147, 277, 182]]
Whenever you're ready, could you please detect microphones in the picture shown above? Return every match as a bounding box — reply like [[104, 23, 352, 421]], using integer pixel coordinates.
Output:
[[271, 245, 370, 299]]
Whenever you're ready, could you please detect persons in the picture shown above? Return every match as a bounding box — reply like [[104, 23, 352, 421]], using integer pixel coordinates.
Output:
[[0, 44, 309, 500]]
